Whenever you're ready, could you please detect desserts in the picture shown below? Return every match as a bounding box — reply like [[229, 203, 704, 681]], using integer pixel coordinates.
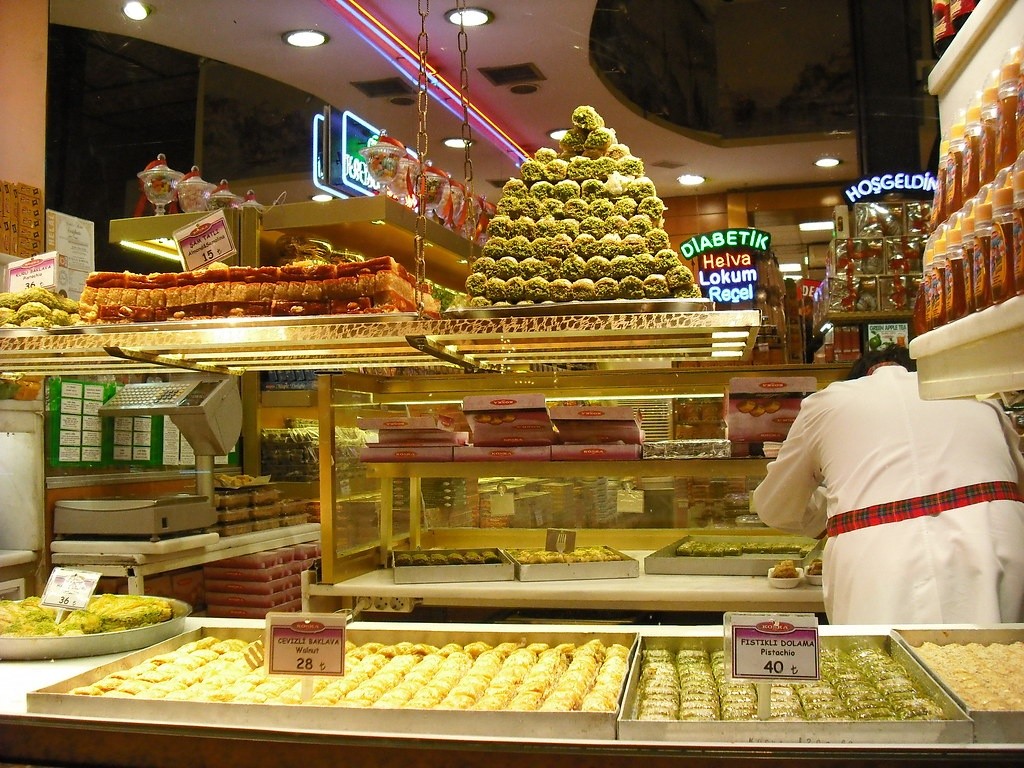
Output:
[[66, 637, 629, 712], [0, 286, 80, 328], [676, 540, 815, 558], [910, 641, 1024, 711], [78, 256, 439, 325], [0, 593, 170, 639], [772, 559, 800, 579], [806, 558, 823, 576], [394, 551, 502, 566], [631, 649, 948, 721], [507, 548, 622, 563], [463, 105, 702, 307]]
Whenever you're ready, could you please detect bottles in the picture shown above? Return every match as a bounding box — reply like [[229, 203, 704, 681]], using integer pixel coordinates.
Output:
[[914, 47, 1024, 336]]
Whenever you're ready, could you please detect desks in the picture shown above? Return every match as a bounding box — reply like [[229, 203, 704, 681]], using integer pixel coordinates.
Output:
[[0, 614, 1024, 766]]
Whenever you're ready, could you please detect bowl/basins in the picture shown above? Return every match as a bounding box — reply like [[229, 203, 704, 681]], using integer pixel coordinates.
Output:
[[763, 442, 783, 458], [805, 566, 822, 586], [768, 567, 803, 588]]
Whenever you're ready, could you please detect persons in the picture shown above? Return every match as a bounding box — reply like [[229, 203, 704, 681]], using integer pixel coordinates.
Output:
[[754, 344, 1024, 624]]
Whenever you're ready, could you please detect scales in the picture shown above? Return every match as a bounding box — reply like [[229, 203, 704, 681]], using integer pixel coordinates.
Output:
[[53, 377, 241, 541]]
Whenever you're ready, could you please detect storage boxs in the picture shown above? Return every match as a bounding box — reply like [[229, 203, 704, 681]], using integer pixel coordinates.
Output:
[[351, 475, 768, 531], [359, 376, 818, 461], [205, 483, 321, 620]]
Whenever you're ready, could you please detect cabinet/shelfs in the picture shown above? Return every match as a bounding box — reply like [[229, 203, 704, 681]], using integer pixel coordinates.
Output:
[[322, 361, 858, 616], [109, 197, 480, 479], [50, 522, 322, 617], [265, 424, 382, 582], [755, 254, 812, 360], [812, 200, 933, 362], [909, 0, 1023, 401]]
